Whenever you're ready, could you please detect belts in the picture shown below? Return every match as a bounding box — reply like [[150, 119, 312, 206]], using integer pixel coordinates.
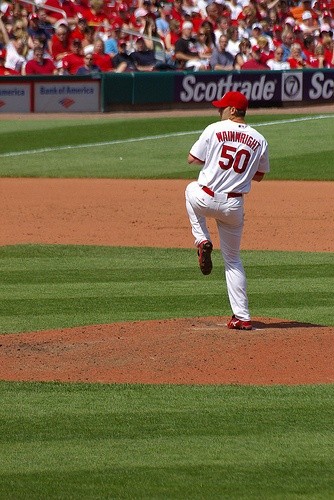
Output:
[[202, 186, 242, 198]]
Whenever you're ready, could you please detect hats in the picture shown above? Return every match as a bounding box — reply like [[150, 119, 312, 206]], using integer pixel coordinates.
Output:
[[322, 10, 333, 20], [211, 90, 248, 111], [301, 10, 312, 20], [252, 22, 263, 31], [135, 7, 149, 17], [118, 39, 126, 46], [252, 45, 261, 52], [182, 21, 193, 29], [135, 36, 143, 42], [285, 17, 295, 26]]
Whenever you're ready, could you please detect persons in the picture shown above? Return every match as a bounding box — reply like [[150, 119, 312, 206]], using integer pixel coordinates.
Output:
[[0, 0, 334, 76], [184, 91, 269, 329]]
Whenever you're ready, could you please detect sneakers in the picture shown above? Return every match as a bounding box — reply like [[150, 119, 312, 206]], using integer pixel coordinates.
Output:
[[227, 315, 253, 330], [196, 240, 214, 275]]
